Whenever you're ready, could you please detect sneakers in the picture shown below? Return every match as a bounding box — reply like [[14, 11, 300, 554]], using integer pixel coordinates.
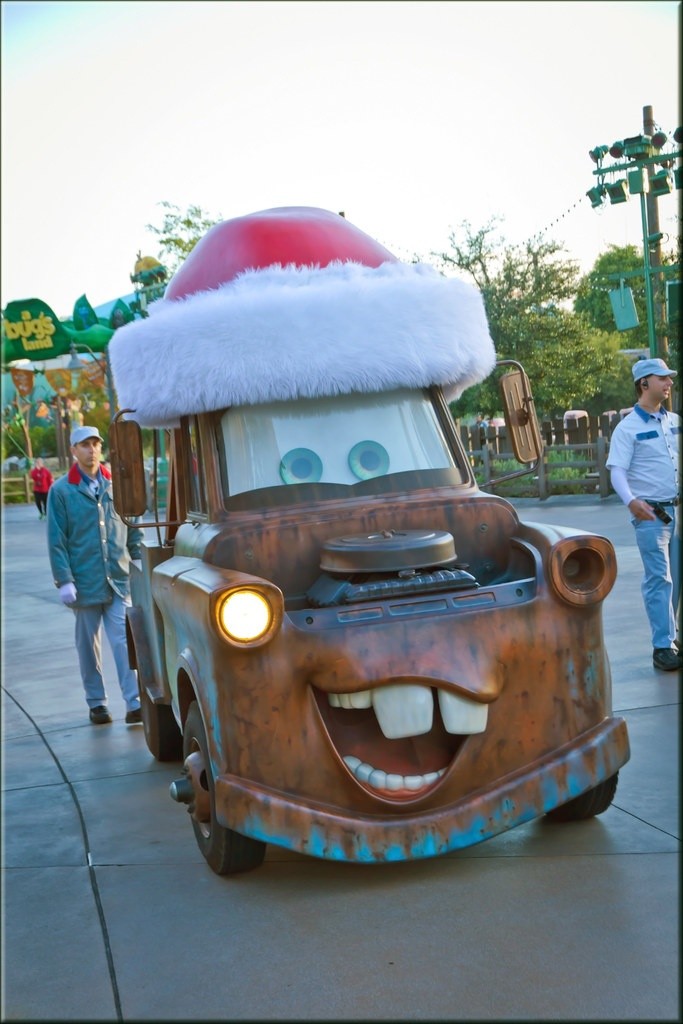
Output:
[[653, 648, 682, 670], [90, 706, 112, 724], [125, 709, 144, 726]]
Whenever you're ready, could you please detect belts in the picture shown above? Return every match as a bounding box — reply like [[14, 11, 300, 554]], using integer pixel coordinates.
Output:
[[648, 498, 682, 506]]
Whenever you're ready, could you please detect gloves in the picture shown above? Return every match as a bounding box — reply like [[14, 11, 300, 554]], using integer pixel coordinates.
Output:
[[59, 582, 78, 604]]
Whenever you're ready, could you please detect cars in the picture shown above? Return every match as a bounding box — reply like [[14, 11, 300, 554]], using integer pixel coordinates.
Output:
[[110, 207, 631, 877]]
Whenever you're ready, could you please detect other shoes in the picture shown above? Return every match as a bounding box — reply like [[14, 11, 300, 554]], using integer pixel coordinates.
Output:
[[39, 514, 44, 520]]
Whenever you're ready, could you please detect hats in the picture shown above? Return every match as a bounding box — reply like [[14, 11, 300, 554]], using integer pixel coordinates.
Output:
[[70, 426, 104, 447], [632, 358, 677, 382]]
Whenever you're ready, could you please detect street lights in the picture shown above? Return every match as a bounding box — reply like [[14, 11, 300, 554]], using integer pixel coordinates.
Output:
[[585, 105, 682, 421]]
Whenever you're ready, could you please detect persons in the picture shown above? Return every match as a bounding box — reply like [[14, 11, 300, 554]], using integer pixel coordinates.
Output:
[[29, 457, 54, 520], [606, 358, 682, 672], [476, 412, 487, 436], [47, 427, 146, 725]]
